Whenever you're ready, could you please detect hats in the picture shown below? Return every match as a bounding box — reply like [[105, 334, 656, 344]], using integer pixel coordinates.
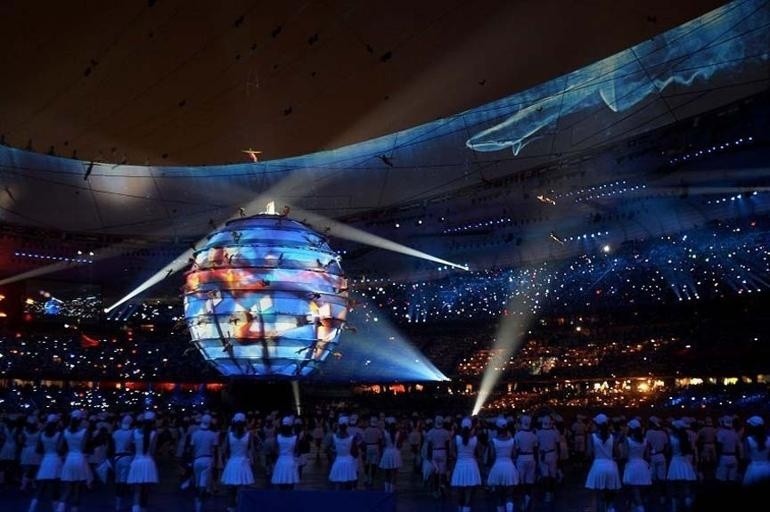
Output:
[[592, 413, 765, 434], [433, 412, 564, 431], [183, 408, 304, 429], [335, 415, 401, 429], [0, 402, 159, 435]]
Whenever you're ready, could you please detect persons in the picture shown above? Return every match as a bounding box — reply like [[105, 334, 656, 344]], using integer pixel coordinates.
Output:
[[1, 135, 769, 408], [1, 410, 769, 510]]
